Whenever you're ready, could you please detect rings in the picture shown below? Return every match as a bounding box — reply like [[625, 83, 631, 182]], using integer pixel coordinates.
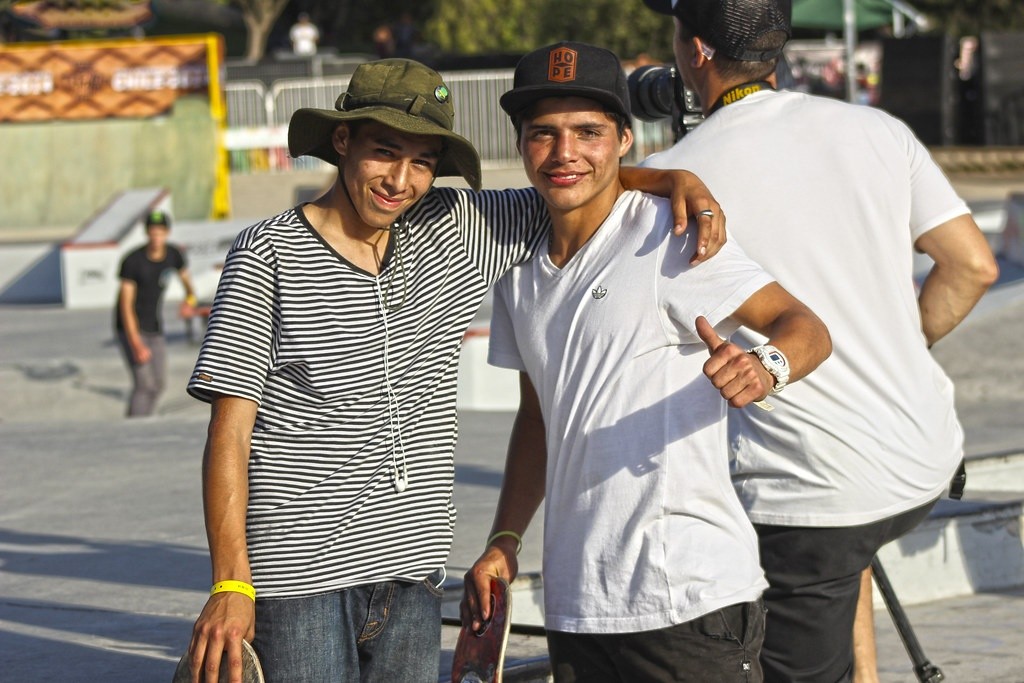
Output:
[[696, 209, 714, 219]]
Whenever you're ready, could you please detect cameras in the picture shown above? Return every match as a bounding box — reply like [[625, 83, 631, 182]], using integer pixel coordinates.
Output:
[[627, 65, 704, 123]]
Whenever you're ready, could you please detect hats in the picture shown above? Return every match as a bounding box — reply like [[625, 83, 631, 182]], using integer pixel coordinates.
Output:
[[145, 210, 170, 229], [288, 59, 482, 193], [498, 42, 632, 129], [646, 0, 792, 61]]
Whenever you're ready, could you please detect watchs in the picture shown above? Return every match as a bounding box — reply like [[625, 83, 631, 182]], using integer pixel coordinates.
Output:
[[730, 325, 790, 396]]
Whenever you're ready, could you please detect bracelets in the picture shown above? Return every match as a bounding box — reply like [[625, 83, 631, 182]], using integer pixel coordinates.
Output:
[[209, 580, 255, 603], [485, 530, 523, 554]]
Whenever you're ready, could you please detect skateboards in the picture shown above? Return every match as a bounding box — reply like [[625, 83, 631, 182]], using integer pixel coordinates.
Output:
[[449, 576, 513, 683], [171, 637, 265, 683]]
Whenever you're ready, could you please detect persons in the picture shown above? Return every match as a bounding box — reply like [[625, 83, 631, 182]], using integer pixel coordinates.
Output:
[[628, 0, 997, 683], [289, 12, 320, 57], [180, 305, 212, 318], [113, 212, 199, 418], [623, 51, 664, 73], [791, 57, 877, 104], [451, 40, 836, 683], [371, 24, 405, 59], [170, 58, 727, 683]]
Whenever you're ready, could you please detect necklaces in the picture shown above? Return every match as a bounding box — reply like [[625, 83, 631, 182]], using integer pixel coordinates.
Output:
[[705, 79, 775, 116]]
